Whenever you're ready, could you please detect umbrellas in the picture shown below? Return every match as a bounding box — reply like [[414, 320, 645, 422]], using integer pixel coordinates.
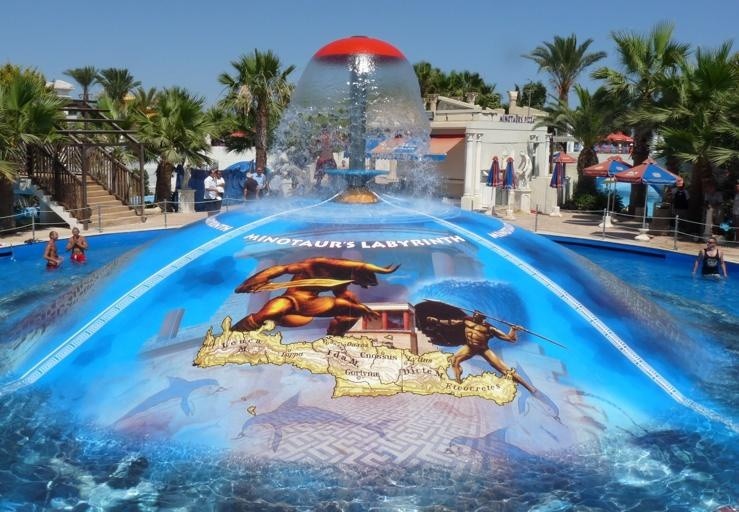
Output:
[[552, 151, 576, 163], [581, 156, 632, 216], [486, 156, 502, 202], [550, 162, 565, 188], [611, 156, 633, 212], [602, 131, 633, 144], [614, 158, 684, 229], [501, 157, 520, 211]]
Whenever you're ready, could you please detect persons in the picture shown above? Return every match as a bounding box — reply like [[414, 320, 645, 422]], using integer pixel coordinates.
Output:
[[203, 169, 218, 218], [693, 236, 728, 280], [213, 169, 226, 213], [668, 181, 739, 243], [242, 164, 308, 203], [311, 128, 345, 189], [43, 231, 65, 270], [66, 227, 88, 265], [425, 309, 537, 394], [516, 151, 532, 191]]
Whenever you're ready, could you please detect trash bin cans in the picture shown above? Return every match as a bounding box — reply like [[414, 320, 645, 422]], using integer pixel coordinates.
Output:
[[177, 188, 196, 213]]
